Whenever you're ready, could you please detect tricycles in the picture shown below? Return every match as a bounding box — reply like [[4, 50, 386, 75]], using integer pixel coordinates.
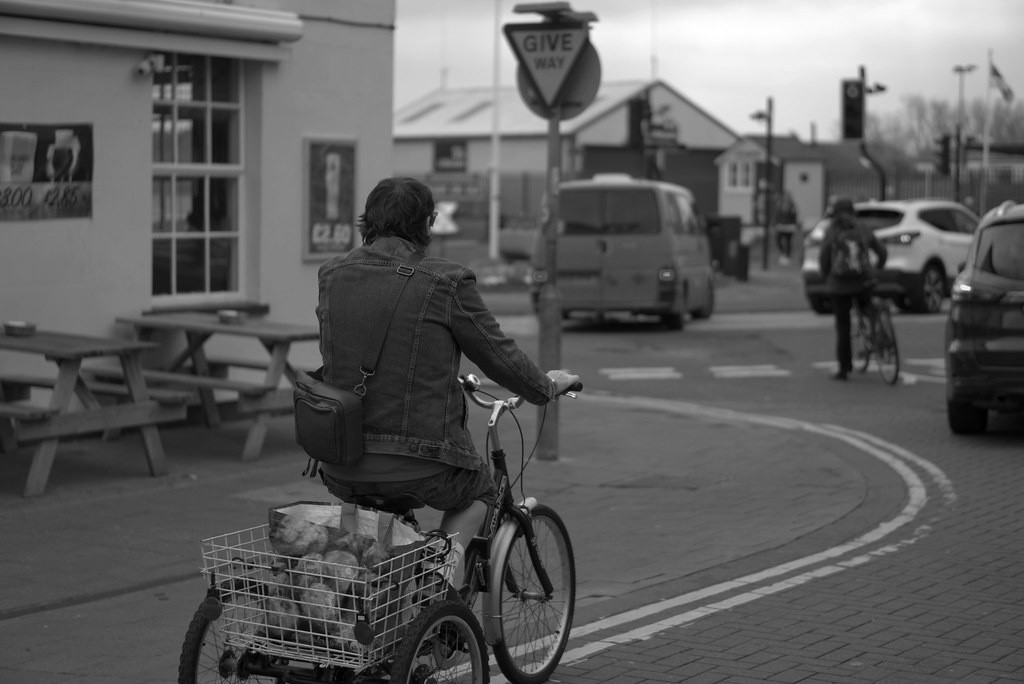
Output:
[[176, 374, 576, 684]]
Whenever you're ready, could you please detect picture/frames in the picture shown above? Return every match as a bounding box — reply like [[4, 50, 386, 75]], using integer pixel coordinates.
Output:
[[300, 134, 360, 263]]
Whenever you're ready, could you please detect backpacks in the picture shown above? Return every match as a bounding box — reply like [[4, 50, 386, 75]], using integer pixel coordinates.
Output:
[[832, 225, 866, 279]]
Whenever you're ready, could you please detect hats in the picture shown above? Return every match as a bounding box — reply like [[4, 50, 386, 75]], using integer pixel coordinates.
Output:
[[834, 200, 854, 217]]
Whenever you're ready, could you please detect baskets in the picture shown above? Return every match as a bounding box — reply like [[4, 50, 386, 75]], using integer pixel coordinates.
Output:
[[198, 525, 460, 671]]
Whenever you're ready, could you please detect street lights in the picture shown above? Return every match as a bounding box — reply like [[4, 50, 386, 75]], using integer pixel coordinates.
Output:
[[952, 65, 976, 201], [750, 113, 770, 268]]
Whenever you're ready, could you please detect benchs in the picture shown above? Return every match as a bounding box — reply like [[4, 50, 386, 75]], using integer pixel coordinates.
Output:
[[0, 357, 321, 419]]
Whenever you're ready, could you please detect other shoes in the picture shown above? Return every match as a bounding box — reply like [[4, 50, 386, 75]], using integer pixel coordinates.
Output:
[[412, 572, 467, 650], [831, 370, 848, 378]]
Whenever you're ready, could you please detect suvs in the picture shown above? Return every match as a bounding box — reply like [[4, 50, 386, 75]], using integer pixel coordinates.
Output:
[[942, 200, 1024, 435]]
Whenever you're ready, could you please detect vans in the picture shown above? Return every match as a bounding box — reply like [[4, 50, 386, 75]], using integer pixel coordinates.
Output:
[[530, 178, 714, 331]]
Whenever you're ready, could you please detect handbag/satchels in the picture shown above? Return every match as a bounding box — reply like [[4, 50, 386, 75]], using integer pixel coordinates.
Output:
[[293, 370, 363, 466], [268, 501, 428, 621]]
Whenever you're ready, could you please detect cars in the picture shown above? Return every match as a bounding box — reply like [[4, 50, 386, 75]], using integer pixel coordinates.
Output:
[[802, 201, 980, 315]]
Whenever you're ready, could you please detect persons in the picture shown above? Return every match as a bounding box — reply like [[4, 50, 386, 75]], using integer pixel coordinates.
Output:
[[316, 179, 579, 654], [820, 198, 887, 378], [774, 193, 797, 263]]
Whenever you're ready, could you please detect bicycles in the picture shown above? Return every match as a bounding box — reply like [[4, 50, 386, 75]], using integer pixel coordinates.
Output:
[[850, 280, 900, 385]]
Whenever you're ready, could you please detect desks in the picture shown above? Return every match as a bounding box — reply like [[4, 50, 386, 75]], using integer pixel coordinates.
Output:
[[0, 330, 172, 497], [115, 310, 320, 460]]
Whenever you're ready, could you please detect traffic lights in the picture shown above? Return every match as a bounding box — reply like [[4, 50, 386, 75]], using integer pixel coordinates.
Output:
[[935, 135, 950, 177]]
